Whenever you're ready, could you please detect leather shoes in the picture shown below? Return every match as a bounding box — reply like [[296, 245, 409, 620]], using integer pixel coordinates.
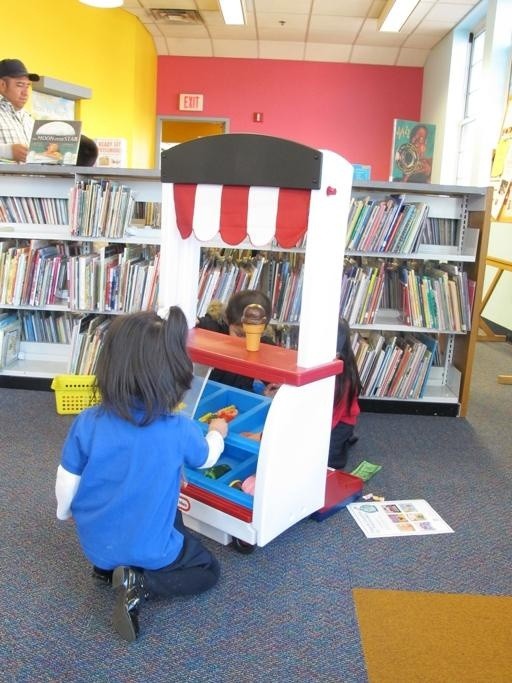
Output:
[[111, 566, 146, 642]]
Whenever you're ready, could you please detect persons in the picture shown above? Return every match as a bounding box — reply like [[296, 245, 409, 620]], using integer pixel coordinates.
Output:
[[199, 295, 277, 393], [331, 320, 361, 469], [401, 125, 432, 183], [54, 304, 226, 646], [0, 60, 41, 165]]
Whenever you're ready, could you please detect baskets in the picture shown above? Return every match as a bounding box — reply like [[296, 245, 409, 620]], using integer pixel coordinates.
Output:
[[51, 374, 101, 415]]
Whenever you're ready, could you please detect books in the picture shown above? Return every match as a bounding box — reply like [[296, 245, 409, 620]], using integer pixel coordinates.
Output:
[[350, 331, 442, 398], [340, 260, 477, 332], [25, 119, 83, 165], [0, 310, 109, 375], [1, 172, 162, 244], [389, 118, 437, 183], [347, 193, 457, 252], [198, 249, 304, 322], [1, 245, 153, 313]]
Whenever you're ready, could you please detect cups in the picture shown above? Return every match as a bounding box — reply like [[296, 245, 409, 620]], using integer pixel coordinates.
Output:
[[243, 324, 265, 352]]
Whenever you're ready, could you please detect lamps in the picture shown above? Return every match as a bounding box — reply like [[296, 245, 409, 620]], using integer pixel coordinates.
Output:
[[219, 0, 249, 25], [80, 1, 124, 9], [377, 0, 420, 34]]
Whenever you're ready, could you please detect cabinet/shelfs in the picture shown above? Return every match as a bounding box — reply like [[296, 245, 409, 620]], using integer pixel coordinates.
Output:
[[0, 163, 494, 417], [154, 132, 353, 549]]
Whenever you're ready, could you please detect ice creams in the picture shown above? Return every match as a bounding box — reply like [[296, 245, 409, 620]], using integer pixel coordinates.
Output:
[[240, 303, 266, 352]]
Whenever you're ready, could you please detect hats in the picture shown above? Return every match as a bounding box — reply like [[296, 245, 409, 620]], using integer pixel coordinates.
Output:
[[0, 59, 40, 82]]
